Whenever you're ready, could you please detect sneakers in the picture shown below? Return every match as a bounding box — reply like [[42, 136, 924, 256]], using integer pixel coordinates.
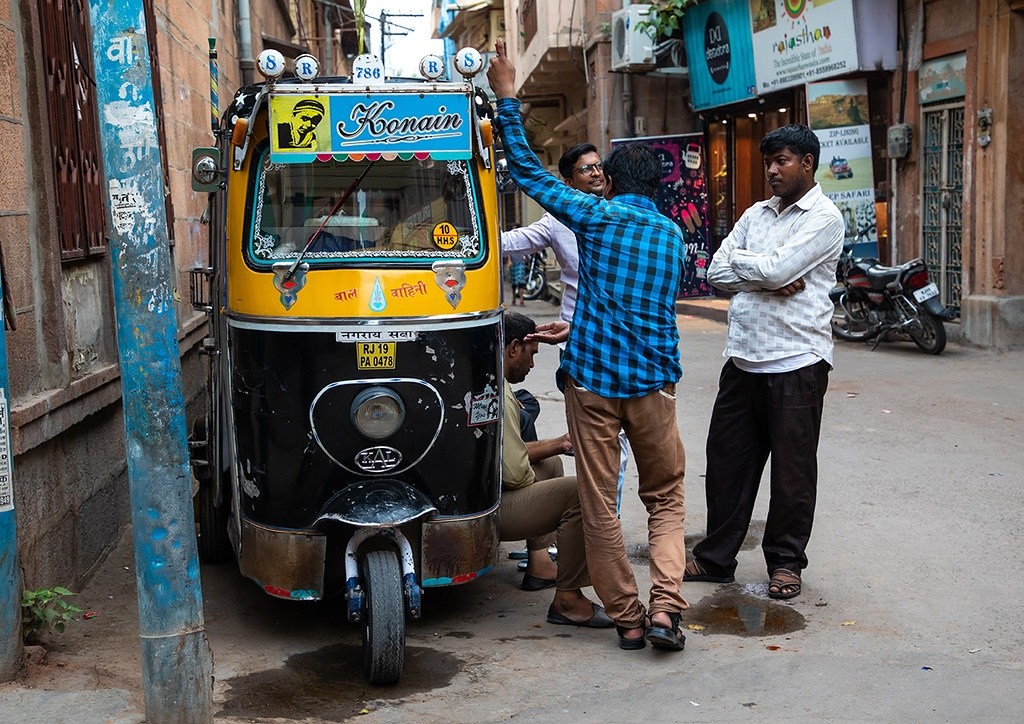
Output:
[[546, 600, 616, 629], [520, 562, 559, 591]]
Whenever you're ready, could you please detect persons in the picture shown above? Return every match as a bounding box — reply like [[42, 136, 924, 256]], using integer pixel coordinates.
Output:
[[498, 143, 629, 527], [684, 124, 846, 601], [486, 35, 692, 653], [496, 310, 625, 631], [513, 388, 541, 444]]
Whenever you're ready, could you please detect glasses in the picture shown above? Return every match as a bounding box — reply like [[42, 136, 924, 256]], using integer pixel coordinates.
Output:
[[571, 161, 605, 175]]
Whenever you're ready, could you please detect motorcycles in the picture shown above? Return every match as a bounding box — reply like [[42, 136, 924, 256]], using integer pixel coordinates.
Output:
[[188, 47, 508, 687], [826, 222, 957, 356], [514, 247, 548, 300]]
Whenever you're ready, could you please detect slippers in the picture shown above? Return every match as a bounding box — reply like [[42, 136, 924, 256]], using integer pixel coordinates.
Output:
[[507, 546, 529, 559], [518, 551, 559, 571]]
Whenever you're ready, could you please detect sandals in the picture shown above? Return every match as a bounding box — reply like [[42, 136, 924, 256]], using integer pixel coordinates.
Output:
[[645, 612, 687, 649], [680, 559, 736, 583], [617, 614, 652, 649], [767, 567, 803, 598]]
